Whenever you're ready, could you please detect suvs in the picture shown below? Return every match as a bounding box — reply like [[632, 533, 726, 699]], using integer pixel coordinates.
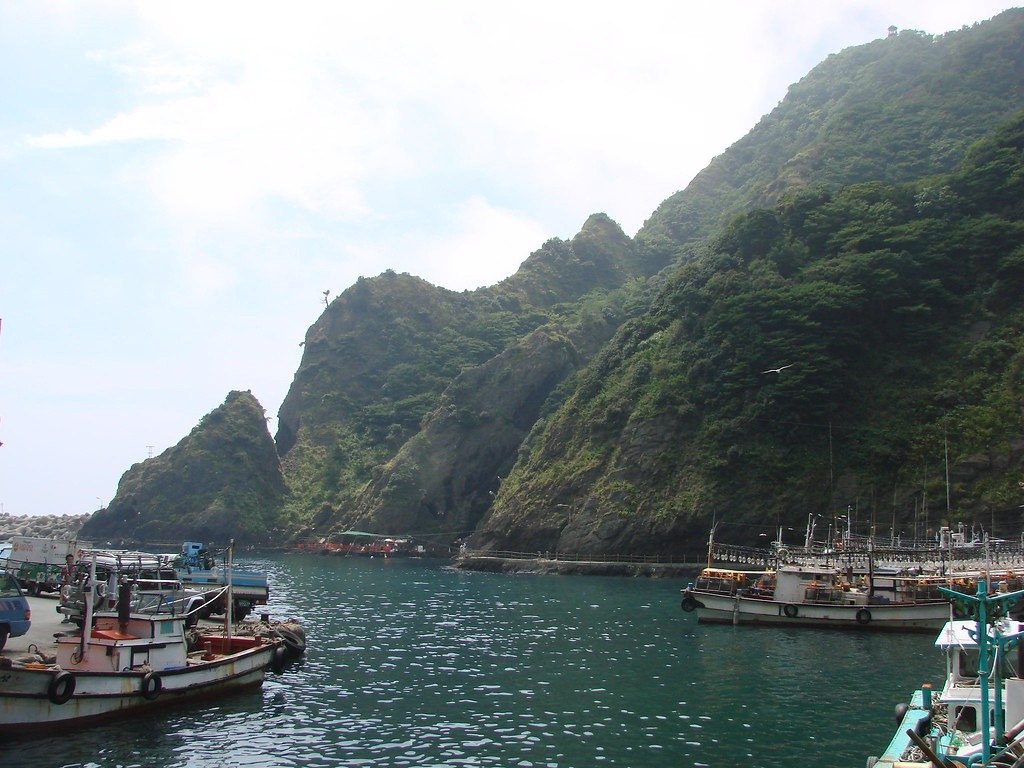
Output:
[[55, 558, 205, 628]]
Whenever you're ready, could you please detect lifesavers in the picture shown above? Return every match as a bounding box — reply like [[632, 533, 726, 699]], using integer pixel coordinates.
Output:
[[856, 608, 872, 625], [953, 605, 964, 618], [275, 629, 307, 665], [141, 672, 162, 700], [48, 670, 77, 705], [680, 596, 697, 612], [784, 603, 798, 618]]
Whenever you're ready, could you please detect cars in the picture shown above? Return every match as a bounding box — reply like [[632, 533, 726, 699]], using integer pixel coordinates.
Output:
[[0, 568, 32, 655]]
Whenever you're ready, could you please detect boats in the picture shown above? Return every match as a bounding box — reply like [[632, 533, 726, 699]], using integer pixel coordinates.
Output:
[[864, 529, 1023, 768], [328, 528, 428, 559], [681, 421, 1023, 636], [0, 539, 285, 733]]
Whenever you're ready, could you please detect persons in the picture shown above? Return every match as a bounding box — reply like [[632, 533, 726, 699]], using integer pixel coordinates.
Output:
[[60, 553, 78, 624], [948, 569, 1022, 589]]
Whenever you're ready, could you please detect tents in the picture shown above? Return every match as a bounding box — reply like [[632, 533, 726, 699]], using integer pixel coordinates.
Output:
[[332, 530, 414, 558]]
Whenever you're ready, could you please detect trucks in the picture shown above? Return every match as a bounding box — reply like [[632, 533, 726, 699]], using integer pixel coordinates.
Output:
[[171, 543, 271, 621], [0, 536, 79, 597]]
